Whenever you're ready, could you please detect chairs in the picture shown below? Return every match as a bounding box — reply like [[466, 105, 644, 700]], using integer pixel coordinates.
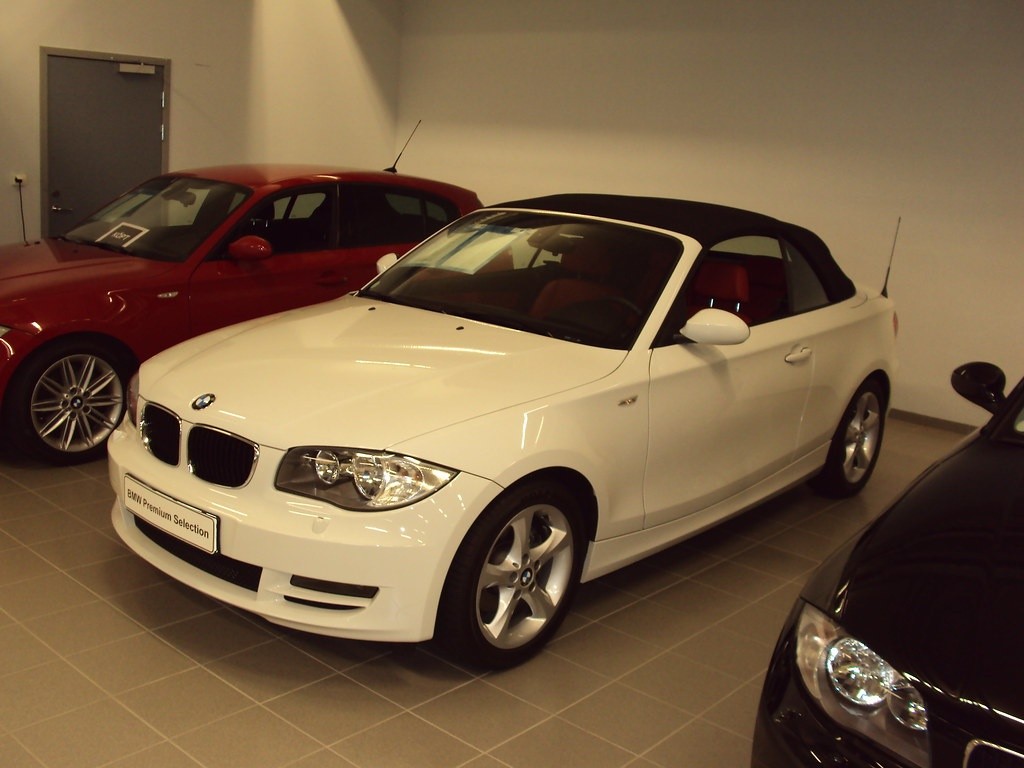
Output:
[[157, 197, 276, 263], [531, 233, 752, 349], [276, 185, 421, 252]]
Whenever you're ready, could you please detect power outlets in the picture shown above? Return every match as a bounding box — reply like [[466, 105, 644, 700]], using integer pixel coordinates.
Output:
[[11, 172, 27, 186]]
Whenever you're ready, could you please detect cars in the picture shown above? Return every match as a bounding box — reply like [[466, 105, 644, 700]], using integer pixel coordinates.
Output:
[[105, 192, 901, 671], [0, 163, 487, 462], [749, 361, 1024, 768]]
